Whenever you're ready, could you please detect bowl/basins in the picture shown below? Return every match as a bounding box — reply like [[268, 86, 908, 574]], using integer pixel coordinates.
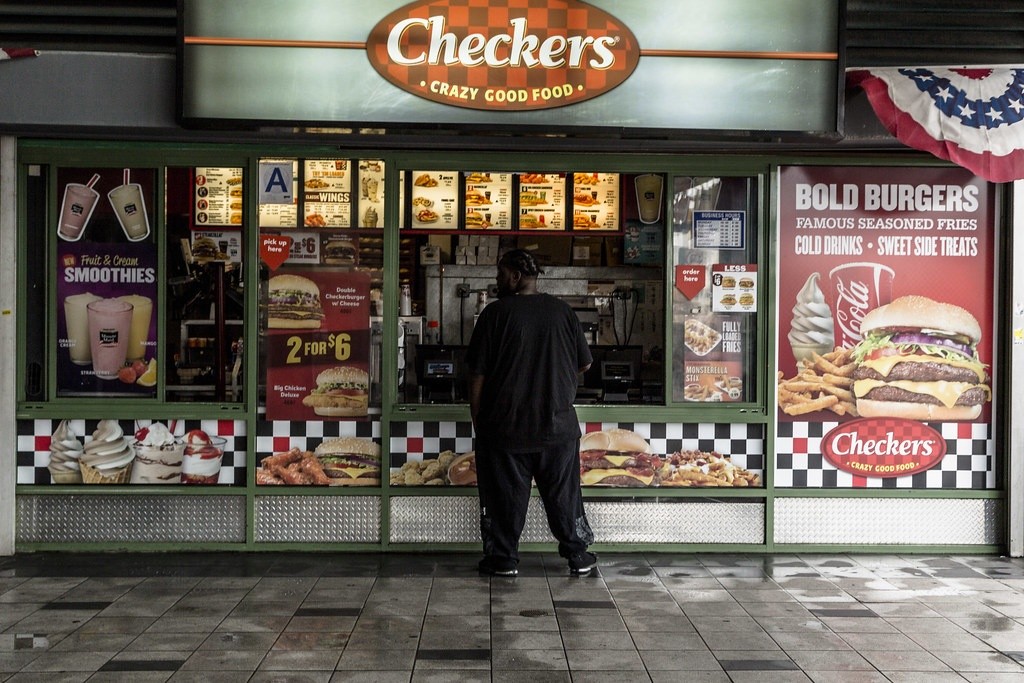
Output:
[[730, 377, 740, 384], [689, 383, 700, 389]]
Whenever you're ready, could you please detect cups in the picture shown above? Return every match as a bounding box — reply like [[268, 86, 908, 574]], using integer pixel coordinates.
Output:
[[130, 439, 183, 485], [176, 435, 227, 485], [828, 261, 897, 350], [65, 295, 103, 366], [120, 293, 151, 366], [59, 184, 95, 239], [633, 173, 664, 224], [110, 187, 147, 241], [88, 299, 133, 380], [675, 177, 722, 227]]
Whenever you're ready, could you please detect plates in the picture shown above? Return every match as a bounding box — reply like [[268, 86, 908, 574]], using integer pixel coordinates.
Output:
[[685, 318, 722, 359], [684, 380, 742, 402]]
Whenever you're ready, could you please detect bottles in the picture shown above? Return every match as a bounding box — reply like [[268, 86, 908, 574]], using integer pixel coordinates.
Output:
[[401, 279, 411, 317], [187, 336, 216, 384]]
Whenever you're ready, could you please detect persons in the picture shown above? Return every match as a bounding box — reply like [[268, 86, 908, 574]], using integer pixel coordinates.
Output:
[[465, 248, 598, 573]]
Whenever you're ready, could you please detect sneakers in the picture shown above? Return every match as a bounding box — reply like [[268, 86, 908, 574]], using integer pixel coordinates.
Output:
[[569, 550, 599, 573], [479, 555, 520, 576]]
